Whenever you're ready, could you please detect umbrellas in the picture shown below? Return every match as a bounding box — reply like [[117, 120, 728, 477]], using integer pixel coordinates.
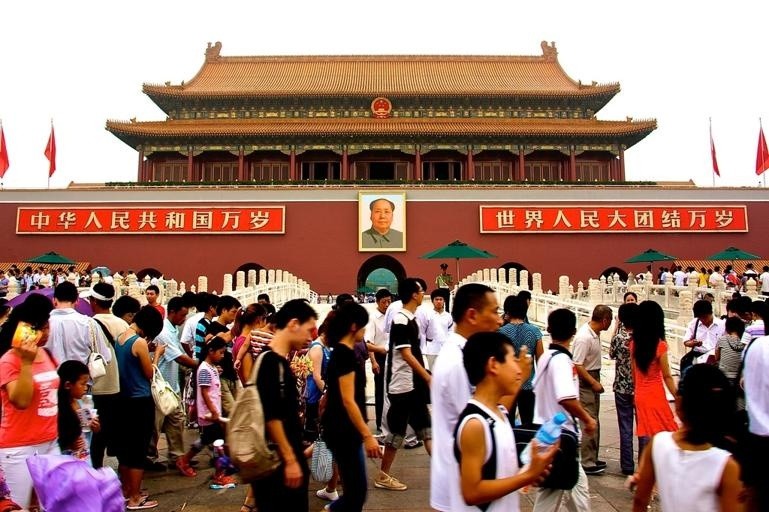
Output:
[[24, 251, 75, 275], [418, 239, 497, 284], [624, 249, 678, 272], [704, 247, 762, 270]]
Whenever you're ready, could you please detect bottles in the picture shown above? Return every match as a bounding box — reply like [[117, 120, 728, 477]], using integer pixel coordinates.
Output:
[[81, 395, 95, 432], [520, 411, 567, 465]]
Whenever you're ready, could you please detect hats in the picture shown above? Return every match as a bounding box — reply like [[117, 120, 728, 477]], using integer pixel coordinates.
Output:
[[79, 283, 114, 302]]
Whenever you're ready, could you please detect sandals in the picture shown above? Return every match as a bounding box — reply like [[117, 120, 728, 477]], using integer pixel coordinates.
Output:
[[240, 503, 259, 512]]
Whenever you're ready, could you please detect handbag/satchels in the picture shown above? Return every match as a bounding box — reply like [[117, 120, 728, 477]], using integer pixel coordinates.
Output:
[[88, 352, 109, 380], [680, 351, 696, 373], [513, 424, 581, 491], [151, 363, 183, 417], [311, 439, 334, 485]]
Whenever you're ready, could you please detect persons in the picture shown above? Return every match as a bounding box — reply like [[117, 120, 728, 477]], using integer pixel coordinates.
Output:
[[0, 264, 769, 512], [363, 199, 403, 247]]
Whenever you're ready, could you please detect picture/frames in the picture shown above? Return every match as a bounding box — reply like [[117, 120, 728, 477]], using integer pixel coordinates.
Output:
[[358, 190, 407, 252]]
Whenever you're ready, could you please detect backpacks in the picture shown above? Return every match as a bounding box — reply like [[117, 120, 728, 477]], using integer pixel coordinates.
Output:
[[226, 351, 286, 481]]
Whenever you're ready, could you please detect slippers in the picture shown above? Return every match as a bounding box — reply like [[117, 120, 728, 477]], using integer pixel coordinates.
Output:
[[127, 500, 159, 510]]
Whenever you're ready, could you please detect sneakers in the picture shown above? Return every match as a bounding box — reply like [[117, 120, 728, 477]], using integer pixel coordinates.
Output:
[[176, 459, 197, 478], [317, 488, 340, 501], [404, 441, 424, 449], [375, 477, 408, 492], [145, 461, 166, 472], [597, 460, 608, 468], [584, 466, 606, 474], [213, 472, 240, 486]]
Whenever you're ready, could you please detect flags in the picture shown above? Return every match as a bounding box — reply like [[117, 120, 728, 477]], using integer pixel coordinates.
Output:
[[712, 141, 720, 176], [0, 128, 9, 177], [44, 128, 56, 178], [756, 129, 769, 175]]
